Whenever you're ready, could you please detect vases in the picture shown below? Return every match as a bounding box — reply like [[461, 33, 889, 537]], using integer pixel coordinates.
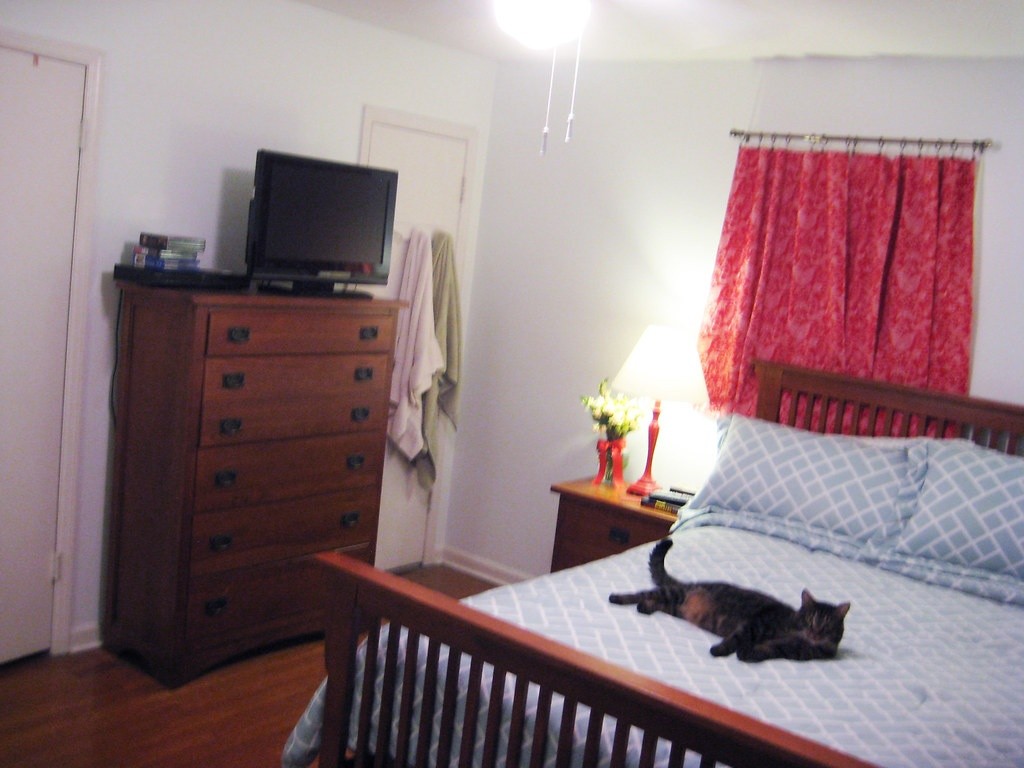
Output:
[[593, 441, 630, 489]]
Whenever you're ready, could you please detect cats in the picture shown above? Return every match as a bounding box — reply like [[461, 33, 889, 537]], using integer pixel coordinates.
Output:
[[606, 536, 851, 664]]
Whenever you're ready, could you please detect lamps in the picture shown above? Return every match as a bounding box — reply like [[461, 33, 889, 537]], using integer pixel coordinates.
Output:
[[495, 0, 594, 157], [627, 361, 682, 498]]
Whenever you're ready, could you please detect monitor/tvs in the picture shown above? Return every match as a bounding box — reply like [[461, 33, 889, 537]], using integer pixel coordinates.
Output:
[[246, 149, 399, 287]]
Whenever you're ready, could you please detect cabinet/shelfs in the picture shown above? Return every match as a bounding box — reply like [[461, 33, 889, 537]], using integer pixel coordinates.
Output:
[[550, 475, 681, 573], [102, 279, 409, 691]]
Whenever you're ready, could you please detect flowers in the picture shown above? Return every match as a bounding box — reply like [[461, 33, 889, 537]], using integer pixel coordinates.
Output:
[[579, 377, 641, 479]]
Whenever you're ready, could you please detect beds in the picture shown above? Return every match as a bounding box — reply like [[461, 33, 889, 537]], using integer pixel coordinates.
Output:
[[277, 521, 1024, 767]]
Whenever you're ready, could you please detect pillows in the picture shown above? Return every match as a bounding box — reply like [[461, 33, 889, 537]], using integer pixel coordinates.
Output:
[[668, 412, 976, 564], [859, 438, 1024, 607]]
